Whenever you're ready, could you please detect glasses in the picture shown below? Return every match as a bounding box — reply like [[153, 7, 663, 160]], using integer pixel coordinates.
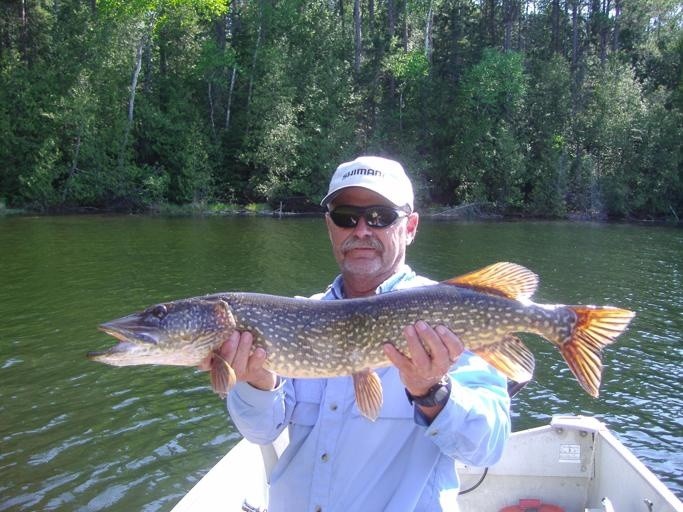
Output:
[[324, 203, 407, 228]]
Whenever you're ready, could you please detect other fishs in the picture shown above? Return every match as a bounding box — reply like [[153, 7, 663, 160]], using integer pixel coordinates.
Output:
[[88, 260, 637, 423]]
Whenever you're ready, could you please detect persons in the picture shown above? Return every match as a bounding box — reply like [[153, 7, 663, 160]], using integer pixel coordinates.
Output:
[[200, 155, 512, 512]]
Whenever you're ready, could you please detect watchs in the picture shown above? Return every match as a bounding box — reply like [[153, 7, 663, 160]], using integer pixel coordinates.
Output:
[[403, 374, 449, 408]]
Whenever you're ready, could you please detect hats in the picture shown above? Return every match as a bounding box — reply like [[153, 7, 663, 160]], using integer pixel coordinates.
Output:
[[318, 154, 416, 215]]
